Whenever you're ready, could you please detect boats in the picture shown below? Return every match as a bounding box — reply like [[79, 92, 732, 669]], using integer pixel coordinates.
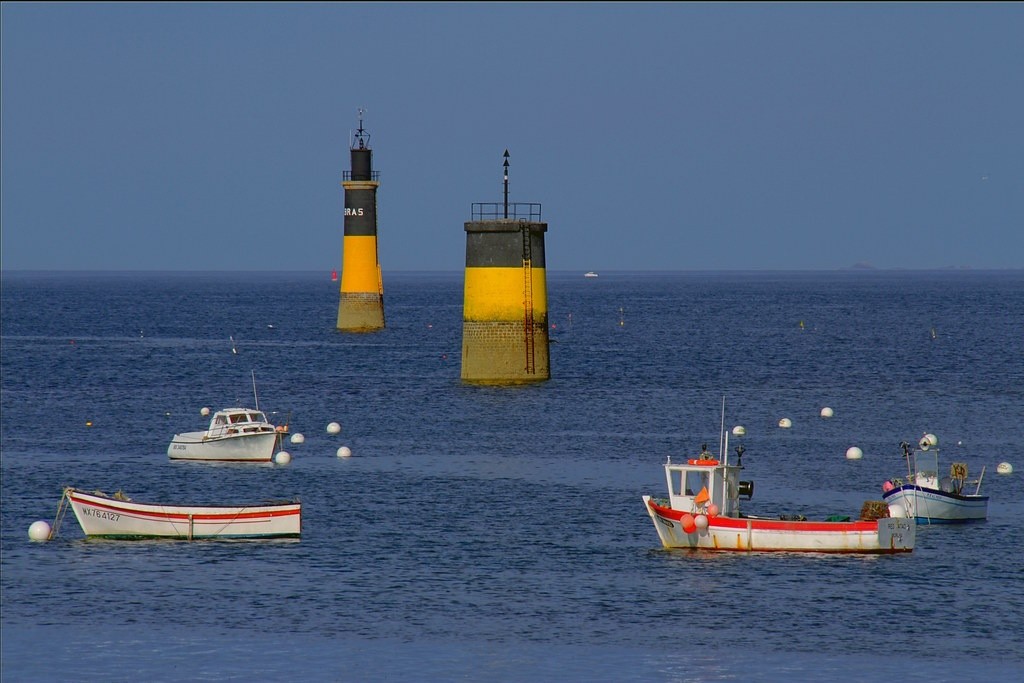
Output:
[[28, 487, 302, 544], [640, 393, 918, 557], [167, 406, 279, 466], [882, 440, 991, 524]]
